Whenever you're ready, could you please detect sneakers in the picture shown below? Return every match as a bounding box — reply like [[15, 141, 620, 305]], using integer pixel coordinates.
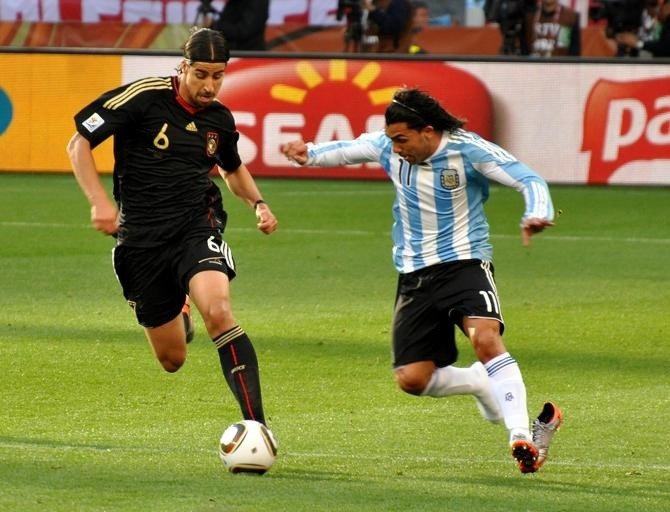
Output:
[[511, 401, 563, 472], [179, 296, 195, 345]]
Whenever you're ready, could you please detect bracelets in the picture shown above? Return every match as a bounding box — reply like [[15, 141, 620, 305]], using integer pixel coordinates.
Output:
[[254, 200, 266, 211]]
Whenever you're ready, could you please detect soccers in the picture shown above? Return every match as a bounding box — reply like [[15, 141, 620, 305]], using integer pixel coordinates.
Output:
[[219, 420, 276, 474]]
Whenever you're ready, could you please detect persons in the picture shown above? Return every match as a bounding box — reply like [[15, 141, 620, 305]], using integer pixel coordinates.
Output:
[[484, 2, 582, 58], [65, 29, 281, 429], [337, 0, 427, 53], [604, 0, 669, 59], [196, 0, 270, 50], [281, 88, 557, 474]]
